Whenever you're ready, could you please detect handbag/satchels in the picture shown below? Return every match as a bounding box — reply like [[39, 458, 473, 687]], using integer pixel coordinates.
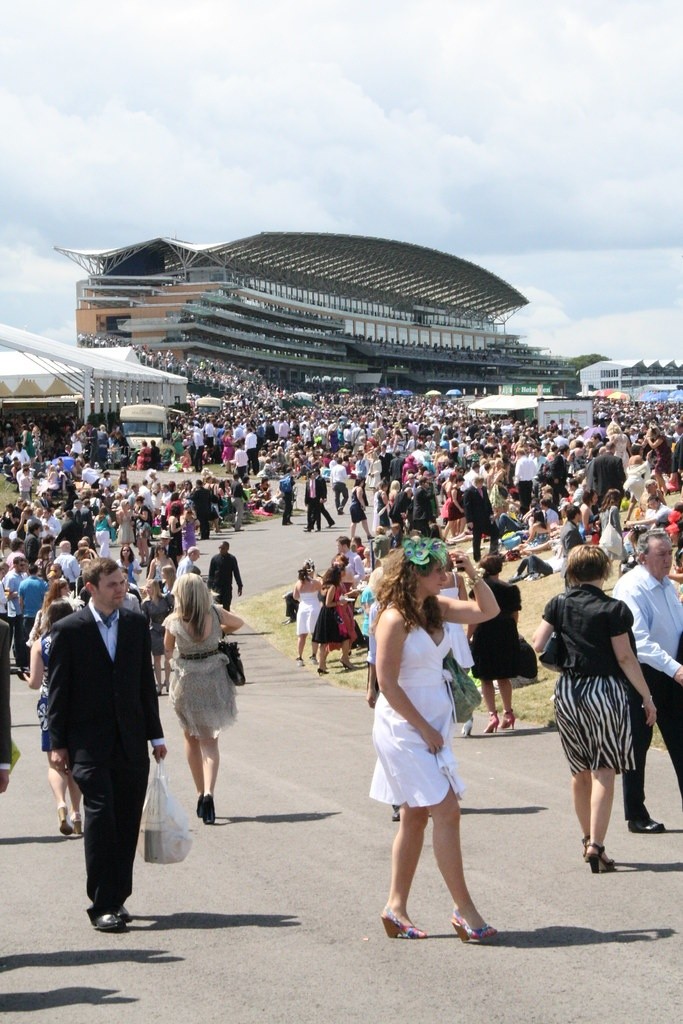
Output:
[[539, 632, 566, 672], [219, 642, 246, 686], [445, 657, 482, 724]]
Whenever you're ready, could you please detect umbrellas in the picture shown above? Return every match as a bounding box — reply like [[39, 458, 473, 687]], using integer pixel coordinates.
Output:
[[387, 387, 393, 393], [338, 388, 350, 393], [652, 392, 668, 403], [635, 392, 654, 401], [371, 386, 379, 392], [667, 389, 683, 403], [379, 387, 390, 394], [607, 391, 628, 403], [445, 389, 462, 395], [425, 390, 442, 398], [403, 390, 414, 395], [595, 389, 613, 397], [393, 389, 403, 395]]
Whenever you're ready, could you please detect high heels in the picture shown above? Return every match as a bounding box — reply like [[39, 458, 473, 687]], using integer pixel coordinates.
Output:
[[70, 812, 82, 835], [484, 711, 499, 733], [501, 709, 515, 730], [581, 835, 616, 874], [197, 795, 215, 826], [340, 658, 354, 669], [452, 909, 496, 943], [380, 909, 428, 940], [317, 668, 329, 675], [461, 720, 473, 737], [57, 805, 73, 835]]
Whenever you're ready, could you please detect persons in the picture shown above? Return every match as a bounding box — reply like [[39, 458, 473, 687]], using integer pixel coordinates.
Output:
[[533, 543, 657, 873], [45, 557, 168, 933], [162, 573, 245, 826], [368, 536, 502, 945], [0, 275, 683, 838]]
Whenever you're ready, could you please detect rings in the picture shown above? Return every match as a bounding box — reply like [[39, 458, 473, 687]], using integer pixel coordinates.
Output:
[[436, 748, 440, 752]]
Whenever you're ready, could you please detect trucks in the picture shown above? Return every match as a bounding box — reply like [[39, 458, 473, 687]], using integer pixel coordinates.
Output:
[[120, 405, 172, 453], [194, 398, 223, 414]]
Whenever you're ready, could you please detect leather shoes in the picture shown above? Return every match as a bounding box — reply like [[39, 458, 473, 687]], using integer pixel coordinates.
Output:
[[629, 813, 664, 833], [93, 905, 132, 930]]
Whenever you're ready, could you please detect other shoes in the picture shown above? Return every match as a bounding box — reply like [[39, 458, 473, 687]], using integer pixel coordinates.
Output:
[[297, 656, 304, 666], [310, 656, 317, 665], [510, 572, 540, 583]]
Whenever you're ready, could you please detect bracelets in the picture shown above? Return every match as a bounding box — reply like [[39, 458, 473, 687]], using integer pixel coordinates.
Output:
[[465, 568, 485, 591], [641, 695, 653, 708]]
